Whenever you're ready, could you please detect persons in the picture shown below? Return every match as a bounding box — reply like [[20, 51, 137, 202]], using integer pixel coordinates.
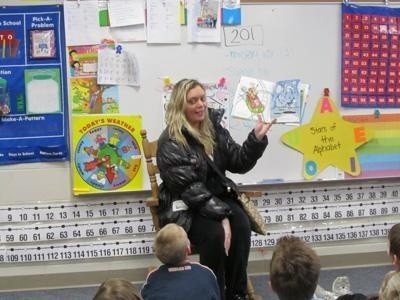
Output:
[[312, 221, 400, 299], [139, 223, 222, 300], [267, 235, 320, 299], [378, 269, 400, 300], [156, 78, 277, 300], [92, 277, 145, 300]]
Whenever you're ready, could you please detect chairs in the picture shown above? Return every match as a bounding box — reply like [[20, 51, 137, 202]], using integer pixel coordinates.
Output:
[[141, 126, 262, 300]]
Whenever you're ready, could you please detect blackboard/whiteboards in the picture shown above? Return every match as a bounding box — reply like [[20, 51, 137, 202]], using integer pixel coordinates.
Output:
[[63, 2, 400, 197]]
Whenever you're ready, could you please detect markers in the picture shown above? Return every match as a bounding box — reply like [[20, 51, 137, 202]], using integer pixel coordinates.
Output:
[[237, 177, 338, 186]]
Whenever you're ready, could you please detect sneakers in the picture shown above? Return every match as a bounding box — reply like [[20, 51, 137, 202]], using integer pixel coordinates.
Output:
[[313, 283, 338, 300], [332, 276, 352, 299]]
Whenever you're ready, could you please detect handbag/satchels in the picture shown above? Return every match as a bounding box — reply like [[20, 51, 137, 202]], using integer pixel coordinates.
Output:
[[238, 192, 268, 236]]
